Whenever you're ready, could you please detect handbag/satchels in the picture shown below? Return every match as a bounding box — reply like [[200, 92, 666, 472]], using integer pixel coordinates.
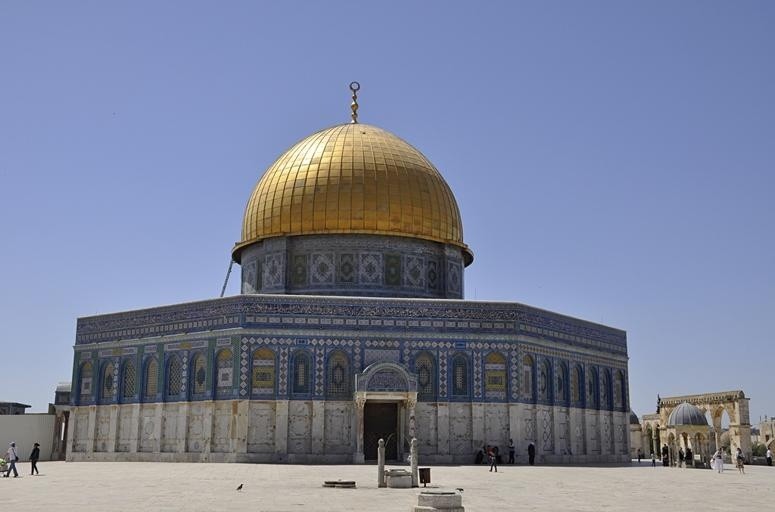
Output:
[[14, 456, 19, 462]]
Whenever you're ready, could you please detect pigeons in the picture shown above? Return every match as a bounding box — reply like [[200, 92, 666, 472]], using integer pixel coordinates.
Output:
[[236, 484, 244, 492], [456, 488, 464, 492]]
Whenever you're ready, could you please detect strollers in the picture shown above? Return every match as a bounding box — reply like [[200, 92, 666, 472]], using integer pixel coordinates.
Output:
[[0, 456, 10, 478]]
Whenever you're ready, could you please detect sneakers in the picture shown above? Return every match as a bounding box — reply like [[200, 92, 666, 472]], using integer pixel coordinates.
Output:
[[3, 474, 9, 477], [489, 470, 497, 472], [30, 471, 39, 475], [13, 474, 18, 478]]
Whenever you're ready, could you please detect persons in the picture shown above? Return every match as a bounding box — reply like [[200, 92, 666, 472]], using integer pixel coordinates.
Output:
[[650, 444, 747, 473], [476, 438, 514, 472], [767, 448, 773, 464], [28, 443, 40, 475], [528, 442, 535, 465], [638, 448, 641, 463], [4, 442, 18, 477]]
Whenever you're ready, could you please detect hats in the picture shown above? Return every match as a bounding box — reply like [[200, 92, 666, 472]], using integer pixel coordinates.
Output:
[[8, 442, 15, 445]]
